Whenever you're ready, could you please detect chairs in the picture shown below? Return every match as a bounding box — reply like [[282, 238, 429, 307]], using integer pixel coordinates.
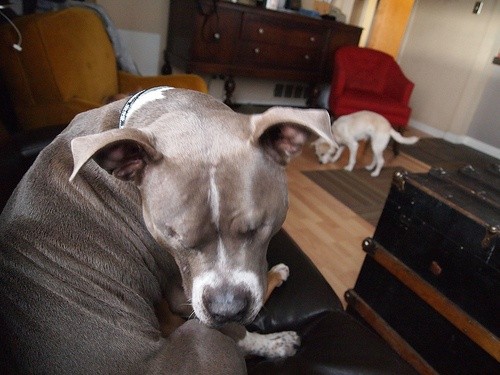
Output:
[[327, 45, 416, 155], [0, 2, 210, 131]]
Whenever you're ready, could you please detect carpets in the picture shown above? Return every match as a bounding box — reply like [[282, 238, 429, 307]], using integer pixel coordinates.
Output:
[[300, 161, 412, 229], [397, 133, 500, 172]]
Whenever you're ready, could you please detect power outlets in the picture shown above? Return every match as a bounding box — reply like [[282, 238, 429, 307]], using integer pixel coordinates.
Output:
[[303, 85, 311, 99], [284, 84, 293, 98], [273, 83, 284, 98], [294, 85, 302, 98]]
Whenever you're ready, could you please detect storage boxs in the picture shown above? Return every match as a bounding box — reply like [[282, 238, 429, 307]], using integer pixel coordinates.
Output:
[[284, 0, 302, 11], [340, 163, 500, 375]]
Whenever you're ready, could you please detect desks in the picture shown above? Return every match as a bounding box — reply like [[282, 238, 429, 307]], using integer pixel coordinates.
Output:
[[161, 0, 365, 111]]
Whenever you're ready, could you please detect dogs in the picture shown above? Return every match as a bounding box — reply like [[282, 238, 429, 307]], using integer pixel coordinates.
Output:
[[1, 85, 340, 375], [309, 110, 419, 176]]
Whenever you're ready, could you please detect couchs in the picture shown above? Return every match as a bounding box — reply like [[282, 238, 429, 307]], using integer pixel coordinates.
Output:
[[0, 126, 420, 375]]
